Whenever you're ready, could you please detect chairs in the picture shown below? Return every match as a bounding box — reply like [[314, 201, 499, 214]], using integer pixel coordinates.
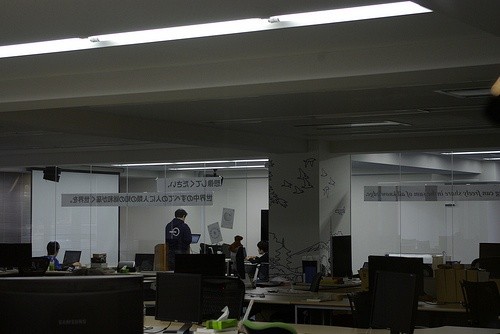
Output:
[[457, 279, 500, 329], [348, 289, 371, 327], [30, 256, 51, 277]]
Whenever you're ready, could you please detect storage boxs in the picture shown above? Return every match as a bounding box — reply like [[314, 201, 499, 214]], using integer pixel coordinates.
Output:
[[91, 253, 107, 269]]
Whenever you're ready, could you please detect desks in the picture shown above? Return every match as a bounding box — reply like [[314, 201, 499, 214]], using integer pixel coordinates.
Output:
[[42, 256, 500, 334]]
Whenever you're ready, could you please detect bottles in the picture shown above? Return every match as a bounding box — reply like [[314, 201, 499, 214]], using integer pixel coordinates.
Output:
[[49, 258, 54, 270]]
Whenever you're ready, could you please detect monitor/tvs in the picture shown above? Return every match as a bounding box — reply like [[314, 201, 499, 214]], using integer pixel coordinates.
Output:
[[136, 254, 155, 269], [151, 272, 201, 333], [0, 242, 32, 270], [62, 250, 82, 265], [0, 274, 147, 334], [191, 233, 201, 245], [173, 253, 225, 277], [368, 253, 424, 334], [478, 242, 500, 281], [301, 260, 318, 285]]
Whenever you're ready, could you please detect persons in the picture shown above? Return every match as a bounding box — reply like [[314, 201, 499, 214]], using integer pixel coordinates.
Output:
[[229, 235, 243, 273], [46, 242, 61, 269], [246, 241, 270, 282], [165, 208, 193, 271]]
